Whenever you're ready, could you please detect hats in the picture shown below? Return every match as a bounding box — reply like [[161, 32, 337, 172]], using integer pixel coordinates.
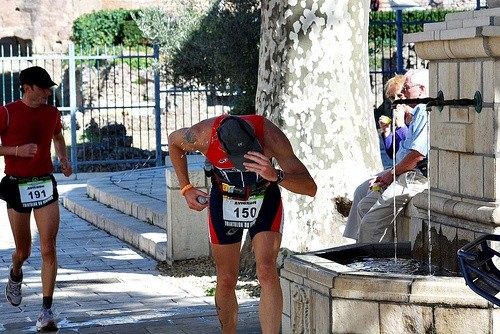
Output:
[[216, 115, 264, 171], [19, 66, 58, 89]]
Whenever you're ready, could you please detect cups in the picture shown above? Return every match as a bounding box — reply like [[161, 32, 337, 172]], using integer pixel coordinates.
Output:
[[371, 180, 380, 191]]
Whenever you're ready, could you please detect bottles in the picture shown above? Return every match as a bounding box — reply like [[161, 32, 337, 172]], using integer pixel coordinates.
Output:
[[197, 196, 207, 204]]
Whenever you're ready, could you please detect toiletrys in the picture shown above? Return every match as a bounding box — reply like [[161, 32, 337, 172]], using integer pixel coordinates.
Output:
[[198, 196, 206, 204]]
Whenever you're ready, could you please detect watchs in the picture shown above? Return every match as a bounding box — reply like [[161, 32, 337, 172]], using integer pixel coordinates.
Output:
[[391, 168, 394, 174], [270, 170, 285, 185]]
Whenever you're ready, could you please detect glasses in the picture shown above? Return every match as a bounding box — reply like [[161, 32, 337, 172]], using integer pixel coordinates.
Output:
[[386, 94, 402, 102], [401, 84, 420, 92]]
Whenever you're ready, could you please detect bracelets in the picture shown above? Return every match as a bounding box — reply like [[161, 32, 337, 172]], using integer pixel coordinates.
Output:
[[182, 184, 193, 195], [15, 146, 18, 156]]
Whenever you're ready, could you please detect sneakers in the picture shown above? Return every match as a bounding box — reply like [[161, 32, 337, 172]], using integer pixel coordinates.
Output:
[[36, 309, 59, 333], [4, 263, 23, 306]]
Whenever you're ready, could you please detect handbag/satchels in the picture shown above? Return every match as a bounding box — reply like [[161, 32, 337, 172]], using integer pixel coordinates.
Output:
[[416, 157, 428, 178]]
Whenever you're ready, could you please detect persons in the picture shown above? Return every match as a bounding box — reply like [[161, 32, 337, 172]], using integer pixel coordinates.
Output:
[[169, 114, 317, 334], [342, 75, 404, 239], [0, 66, 72, 332], [357, 68, 429, 243]]
